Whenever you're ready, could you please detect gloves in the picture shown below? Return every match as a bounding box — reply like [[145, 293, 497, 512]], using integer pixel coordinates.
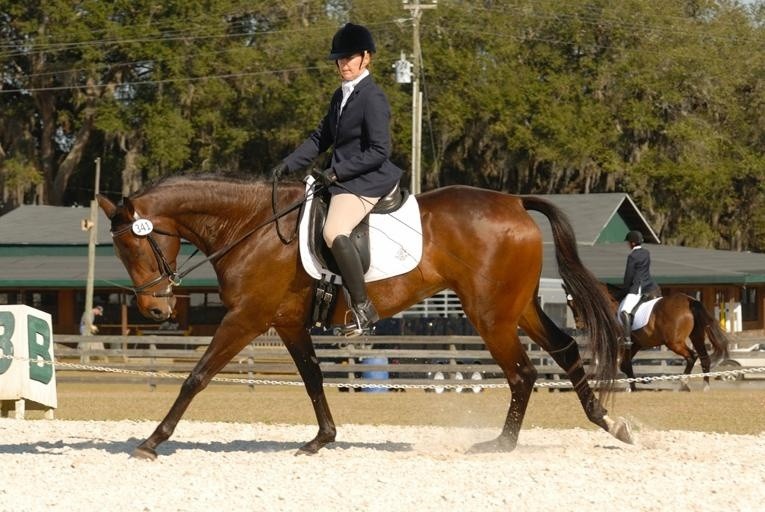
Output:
[[317, 167, 338, 187], [270, 163, 288, 181]]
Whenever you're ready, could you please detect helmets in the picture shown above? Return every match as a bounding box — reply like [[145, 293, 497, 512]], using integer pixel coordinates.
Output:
[[622, 230, 646, 245], [327, 24, 375, 59]]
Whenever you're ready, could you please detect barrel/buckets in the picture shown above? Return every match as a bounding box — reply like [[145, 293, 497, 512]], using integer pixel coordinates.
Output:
[[360, 351, 389, 392]]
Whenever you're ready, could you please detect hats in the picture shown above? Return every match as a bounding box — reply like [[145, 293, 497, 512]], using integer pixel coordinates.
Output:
[[98, 305, 104, 316]]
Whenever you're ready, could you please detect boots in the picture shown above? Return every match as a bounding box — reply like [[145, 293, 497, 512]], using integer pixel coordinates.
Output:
[[330, 235, 381, 338], [617, 310, 635, 349]]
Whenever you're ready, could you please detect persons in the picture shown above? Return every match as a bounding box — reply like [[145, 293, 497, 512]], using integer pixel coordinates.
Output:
[[272, 24, 407, 338], [158, 308, 180, 330], [618, 231, 658, 344], [79, 305, 103, 364]]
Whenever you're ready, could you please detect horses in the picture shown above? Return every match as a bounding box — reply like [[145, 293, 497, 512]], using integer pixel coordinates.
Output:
[[95, 165, 637, 463], [560, 281, 730, 393]]
